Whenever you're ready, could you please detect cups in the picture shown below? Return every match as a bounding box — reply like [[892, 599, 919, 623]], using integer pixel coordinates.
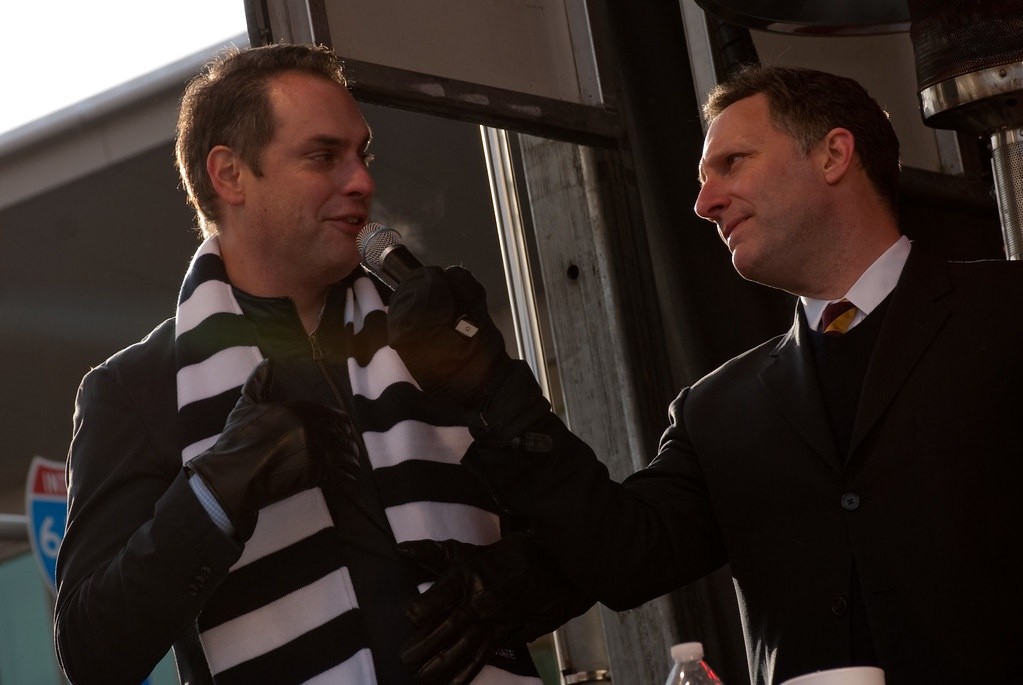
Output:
[[781, 666, 885, 684]]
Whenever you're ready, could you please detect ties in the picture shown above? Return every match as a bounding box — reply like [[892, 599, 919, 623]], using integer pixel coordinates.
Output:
[[819, 298, 858, 337]]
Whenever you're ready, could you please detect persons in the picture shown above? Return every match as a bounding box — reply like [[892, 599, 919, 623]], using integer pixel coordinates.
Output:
[[385, 67, 1023, 684], [51, 40, 605, 684]]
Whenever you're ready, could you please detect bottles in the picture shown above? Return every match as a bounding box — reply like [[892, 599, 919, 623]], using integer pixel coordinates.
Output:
[[666, 641, 725, 685]]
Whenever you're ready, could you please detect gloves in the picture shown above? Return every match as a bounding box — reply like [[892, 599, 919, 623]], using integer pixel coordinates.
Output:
[[381, 264, 552, 450], [181, 361, 362, 545], [392, 528, 580, 685]]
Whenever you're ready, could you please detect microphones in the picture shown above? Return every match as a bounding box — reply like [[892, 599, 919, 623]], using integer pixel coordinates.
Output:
[[356, 223, 480, 340]]
